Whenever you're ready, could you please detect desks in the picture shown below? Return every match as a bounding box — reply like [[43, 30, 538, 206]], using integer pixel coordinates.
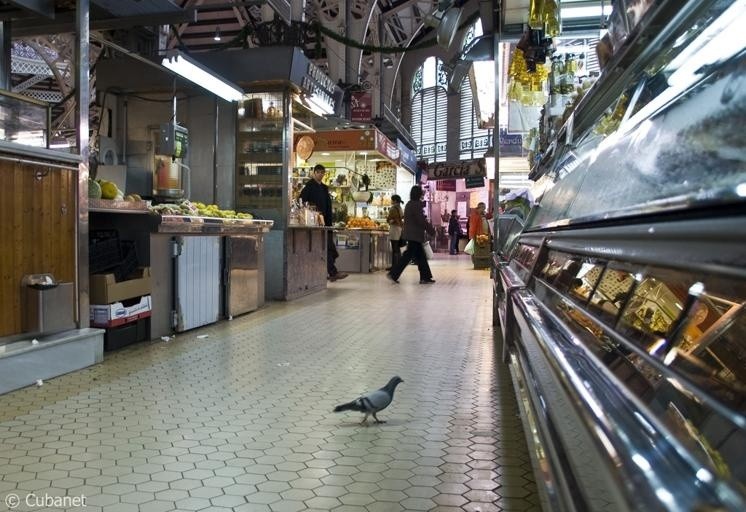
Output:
[[266, 225, 337, 301], [334, 227, 389, 273], [88, 206, 275, 341], [1, 138, 83, 342]]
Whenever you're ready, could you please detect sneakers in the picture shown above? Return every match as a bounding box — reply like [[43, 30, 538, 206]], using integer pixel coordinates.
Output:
[[421, 278, 435, 282], [389, 272, 399, 283], [331, 273, 348, 282]]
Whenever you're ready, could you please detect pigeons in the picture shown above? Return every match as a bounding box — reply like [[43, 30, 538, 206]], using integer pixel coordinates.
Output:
[[333, 376, 404, 425]]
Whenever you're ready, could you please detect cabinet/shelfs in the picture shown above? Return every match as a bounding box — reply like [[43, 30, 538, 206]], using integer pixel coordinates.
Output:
[[238, 88, 290, 225], [293, 166, 363, 225], [494, 0, 746, 511]]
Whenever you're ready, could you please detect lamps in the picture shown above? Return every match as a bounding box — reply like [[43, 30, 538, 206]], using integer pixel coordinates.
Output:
[[160, 48, 244, 103], [211, 12, 222, 40]]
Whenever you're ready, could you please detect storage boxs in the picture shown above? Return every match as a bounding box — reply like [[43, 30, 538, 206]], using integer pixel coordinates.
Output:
[[91, 328, 153, 351], [90, 266, 150, 304], [90, 296, 152, 328]]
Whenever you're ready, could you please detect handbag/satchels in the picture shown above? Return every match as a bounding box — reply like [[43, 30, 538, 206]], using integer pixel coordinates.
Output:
[[399, 231, 406, 247], [423, 241, 433, 259]]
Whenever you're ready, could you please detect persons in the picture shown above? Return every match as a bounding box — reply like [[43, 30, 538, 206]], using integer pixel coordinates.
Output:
[[447, 202, 494, 255], [297, 164, 348, 282], [385, 194, 404, 271], [387, 186, 436, 284]]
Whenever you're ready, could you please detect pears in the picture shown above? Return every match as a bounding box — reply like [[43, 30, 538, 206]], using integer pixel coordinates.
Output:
[[347, 211, 378, 229]]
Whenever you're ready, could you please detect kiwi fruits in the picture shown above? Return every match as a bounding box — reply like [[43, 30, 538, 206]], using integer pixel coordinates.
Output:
[[132, 193, 141, 203], [124, 195, 135, 203]]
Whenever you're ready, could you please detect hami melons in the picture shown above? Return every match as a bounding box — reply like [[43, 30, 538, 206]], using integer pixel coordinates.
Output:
[[87, 179, 103, 200]]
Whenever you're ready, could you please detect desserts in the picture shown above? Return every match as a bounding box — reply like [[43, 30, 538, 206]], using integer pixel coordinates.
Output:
[[100, 182, 119, 200]]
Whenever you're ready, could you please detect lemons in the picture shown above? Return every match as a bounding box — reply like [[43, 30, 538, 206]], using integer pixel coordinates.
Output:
[[191, 200, 255, 220]]
